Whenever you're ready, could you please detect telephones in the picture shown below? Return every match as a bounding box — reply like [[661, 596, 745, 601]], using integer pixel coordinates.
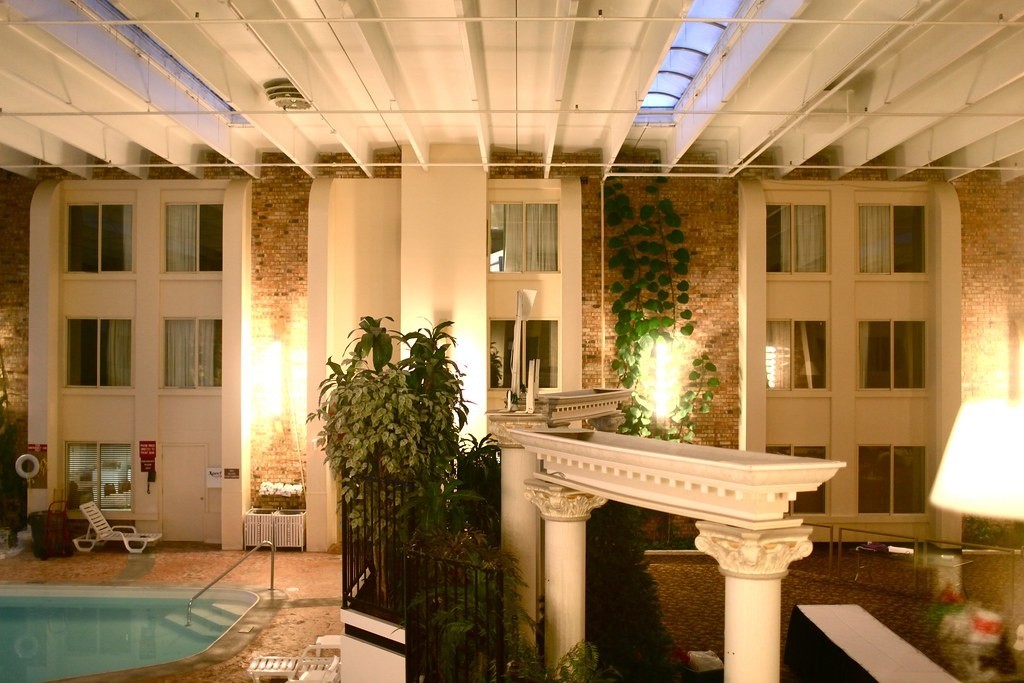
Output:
[[147, 470, 156, 482]]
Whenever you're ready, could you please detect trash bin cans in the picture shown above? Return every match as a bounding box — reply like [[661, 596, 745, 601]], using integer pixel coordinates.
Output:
[[26, 509, 65, 557]]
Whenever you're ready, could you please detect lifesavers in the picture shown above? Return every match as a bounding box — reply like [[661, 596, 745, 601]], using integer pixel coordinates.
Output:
[[15, 453, 40, 480]]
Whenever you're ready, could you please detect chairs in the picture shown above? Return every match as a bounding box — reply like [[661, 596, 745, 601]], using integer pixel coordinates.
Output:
[[246, 642, 341, 683], [72, 501, 163, 554]]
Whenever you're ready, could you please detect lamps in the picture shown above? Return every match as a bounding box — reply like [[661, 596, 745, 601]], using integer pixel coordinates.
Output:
[[931, 398, 1024, 520]]
